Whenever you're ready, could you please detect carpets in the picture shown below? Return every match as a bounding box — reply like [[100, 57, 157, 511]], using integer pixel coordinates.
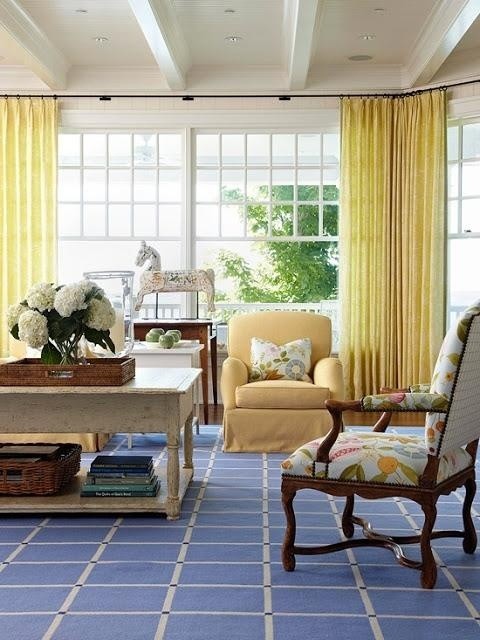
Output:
[[0, 422, 480, 640]]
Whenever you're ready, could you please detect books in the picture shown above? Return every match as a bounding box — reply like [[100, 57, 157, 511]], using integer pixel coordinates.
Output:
[[80, 455, 160, 496]]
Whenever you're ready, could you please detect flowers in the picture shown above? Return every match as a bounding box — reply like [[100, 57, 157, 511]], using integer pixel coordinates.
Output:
[[4, 277, 118, 365]]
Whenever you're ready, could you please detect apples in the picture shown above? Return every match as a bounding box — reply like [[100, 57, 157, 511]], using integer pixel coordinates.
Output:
[[145, 328, 181, 349]]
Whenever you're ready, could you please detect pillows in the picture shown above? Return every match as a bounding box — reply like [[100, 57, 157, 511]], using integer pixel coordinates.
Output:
[[248, 337, 312, 383]]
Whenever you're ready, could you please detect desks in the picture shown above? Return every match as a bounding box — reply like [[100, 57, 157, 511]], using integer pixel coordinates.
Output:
[[133, 318, 214, 424], [132, 337, 204, 439], [0, 368, 204, 520]]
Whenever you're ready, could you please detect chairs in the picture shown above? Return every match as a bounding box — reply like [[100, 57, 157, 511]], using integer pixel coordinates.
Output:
[[279, 300, 480, 589], [220, 311, 344, 453]]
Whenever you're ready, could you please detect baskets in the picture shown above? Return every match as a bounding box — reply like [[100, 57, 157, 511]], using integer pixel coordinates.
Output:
[[0, 443, 82, 499]]
